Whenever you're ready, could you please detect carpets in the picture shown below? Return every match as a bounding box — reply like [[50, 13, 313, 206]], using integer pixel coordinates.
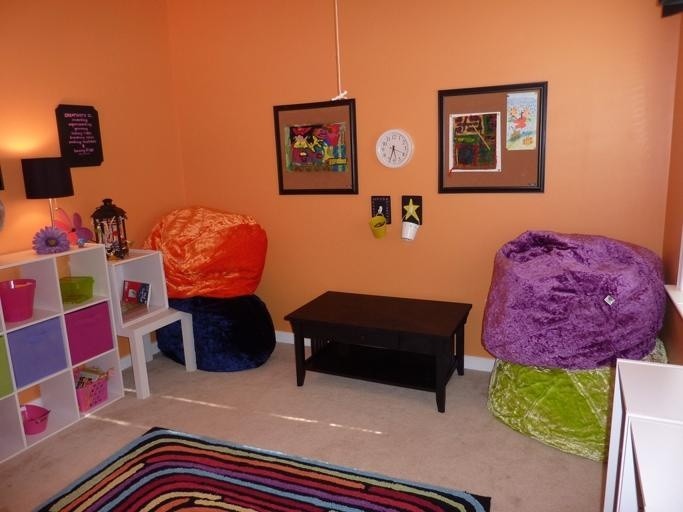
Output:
[[33, 426, 491, 511]]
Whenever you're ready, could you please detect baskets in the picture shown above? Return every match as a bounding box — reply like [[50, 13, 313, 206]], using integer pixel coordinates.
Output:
[[75, 370, 113, 410]]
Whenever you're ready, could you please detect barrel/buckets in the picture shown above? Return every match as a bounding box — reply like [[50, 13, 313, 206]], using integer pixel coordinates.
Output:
[[0, 278, 36, 323], [401, 214, 419, 241], [369, 215, 387, 238]]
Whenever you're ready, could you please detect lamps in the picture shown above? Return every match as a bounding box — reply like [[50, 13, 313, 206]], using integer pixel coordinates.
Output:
[[21, 157, 74, 228]]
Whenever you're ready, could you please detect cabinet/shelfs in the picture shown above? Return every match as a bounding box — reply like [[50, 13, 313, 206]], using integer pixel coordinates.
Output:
[[1, 238, 124, 462], [602, 357, 683, 512]]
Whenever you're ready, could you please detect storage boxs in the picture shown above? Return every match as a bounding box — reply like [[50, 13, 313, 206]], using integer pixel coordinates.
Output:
[[107, 250, 170, 328]]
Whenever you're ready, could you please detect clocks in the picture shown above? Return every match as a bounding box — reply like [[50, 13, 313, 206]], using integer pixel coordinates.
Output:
[[375, 128, 415, 169]]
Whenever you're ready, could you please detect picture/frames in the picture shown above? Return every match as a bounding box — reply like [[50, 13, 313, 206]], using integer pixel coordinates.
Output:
[[273, 98, 358, 194], [438, 81, 549, 194]]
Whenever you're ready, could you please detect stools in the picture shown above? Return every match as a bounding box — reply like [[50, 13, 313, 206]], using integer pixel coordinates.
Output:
[[114, 306, 197, 400]]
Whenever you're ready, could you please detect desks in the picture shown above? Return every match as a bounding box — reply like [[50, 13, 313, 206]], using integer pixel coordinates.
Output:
[[283, 289, 473, 413]]
